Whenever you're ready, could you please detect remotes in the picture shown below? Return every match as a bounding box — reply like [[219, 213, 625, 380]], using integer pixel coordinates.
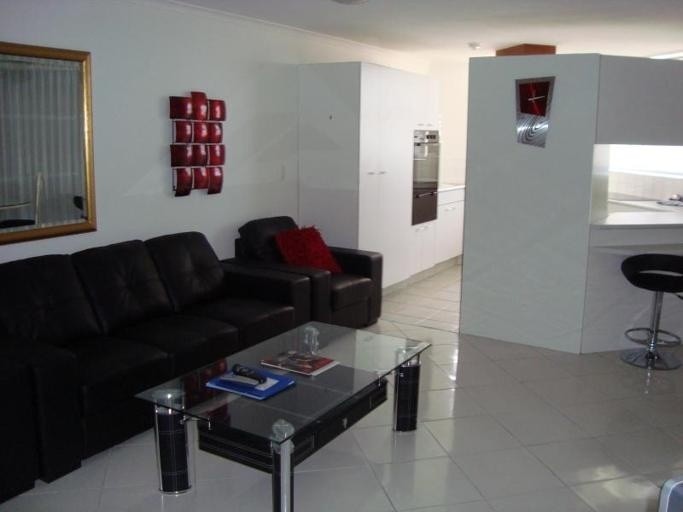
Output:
[[220, 374, 259, 387], [232, 364, 266, 384]]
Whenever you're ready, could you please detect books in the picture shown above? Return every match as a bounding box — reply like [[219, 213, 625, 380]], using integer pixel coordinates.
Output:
[[260, 349, 334, 376]]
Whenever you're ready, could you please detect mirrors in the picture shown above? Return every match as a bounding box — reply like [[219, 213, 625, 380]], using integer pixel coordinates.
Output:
[[0, 42, 97, 245]]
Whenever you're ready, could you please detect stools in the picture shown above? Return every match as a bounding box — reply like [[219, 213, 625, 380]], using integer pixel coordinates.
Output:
[[620, 253, 683, 370]]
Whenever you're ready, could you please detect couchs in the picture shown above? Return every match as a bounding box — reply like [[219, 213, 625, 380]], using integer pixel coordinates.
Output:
[[1, 232, 309, 505]]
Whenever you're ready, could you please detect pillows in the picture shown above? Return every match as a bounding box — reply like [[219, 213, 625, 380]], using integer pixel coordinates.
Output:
[[274, 225, 342, 274]]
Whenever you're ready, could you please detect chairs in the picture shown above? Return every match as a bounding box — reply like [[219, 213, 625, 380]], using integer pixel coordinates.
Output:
[[235, 215, 384, 329], [0, 172, 43, 231]]
